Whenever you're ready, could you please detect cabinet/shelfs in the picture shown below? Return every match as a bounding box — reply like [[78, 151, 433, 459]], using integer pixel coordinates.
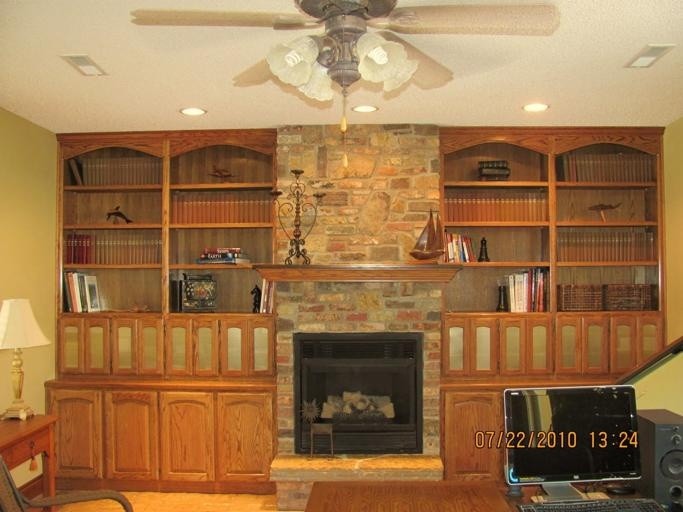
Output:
[[438, 126, 667, 485], [46, 127, 273, 484]]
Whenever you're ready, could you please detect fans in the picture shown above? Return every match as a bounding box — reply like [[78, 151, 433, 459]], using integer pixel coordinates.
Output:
[[131, 0, 562, 92]]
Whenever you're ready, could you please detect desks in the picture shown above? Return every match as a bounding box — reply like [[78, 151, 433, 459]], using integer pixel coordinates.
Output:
[[0, 415, 58, 512], [299, 482, 547, 512]]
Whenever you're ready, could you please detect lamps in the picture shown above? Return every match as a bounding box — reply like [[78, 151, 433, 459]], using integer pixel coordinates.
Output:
[[260, 15, 423, 133], [1, 299, 51, 422]]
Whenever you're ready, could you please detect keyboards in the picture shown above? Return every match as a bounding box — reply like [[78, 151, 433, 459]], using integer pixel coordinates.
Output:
[[516, 497, 666, 512]]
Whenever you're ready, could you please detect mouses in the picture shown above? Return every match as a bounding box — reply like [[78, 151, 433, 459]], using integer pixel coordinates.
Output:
[[668, 500, 682, 512]]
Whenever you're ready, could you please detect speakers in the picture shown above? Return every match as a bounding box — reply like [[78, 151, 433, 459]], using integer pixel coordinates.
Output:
[[636, 409, 683, 512]]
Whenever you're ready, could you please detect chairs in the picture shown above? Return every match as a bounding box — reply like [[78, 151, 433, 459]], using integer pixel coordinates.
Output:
[[0, 453, 133, 512]]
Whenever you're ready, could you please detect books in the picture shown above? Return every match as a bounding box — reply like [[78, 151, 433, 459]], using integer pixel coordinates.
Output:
[[196, 247, 251, 265], [259, 278, 275, 314], [170, 190, 272, 224], [444, 230, 491, 262], [556, 228, 654, 263], [443, 197, 547, 222], [477, 160, 511, 181], [496, 270, 657, 311], [70, 155, 162, 186], [65, 229, 162, 264], [63, 272, 101, 314], [562, 152, 653, 181]]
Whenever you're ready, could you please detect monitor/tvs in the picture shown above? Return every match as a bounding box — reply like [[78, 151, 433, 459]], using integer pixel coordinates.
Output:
[[503, 385, 642, 500]]
